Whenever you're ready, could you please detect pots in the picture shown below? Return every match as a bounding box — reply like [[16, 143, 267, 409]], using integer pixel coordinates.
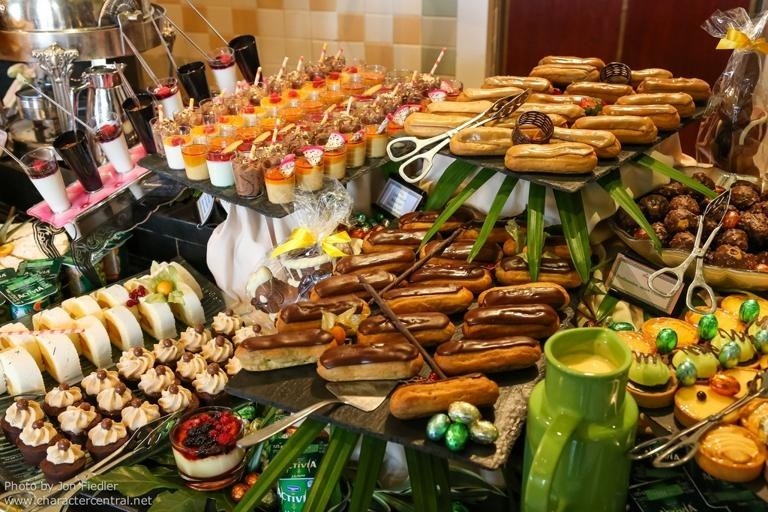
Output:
[[0, 85, 95, 159]]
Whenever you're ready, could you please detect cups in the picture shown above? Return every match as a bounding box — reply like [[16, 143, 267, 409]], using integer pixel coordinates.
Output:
[[17, 145, 72, 215], [121, 91, 156, 155], [85, 111, 136, 175], [146, 35, 464, 205], [52, 129, 104, 194]]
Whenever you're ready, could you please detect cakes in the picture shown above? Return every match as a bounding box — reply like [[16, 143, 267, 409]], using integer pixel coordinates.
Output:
[[614, 173, 768, 273], [617, 294, 768, 482], [0, 261, 267, 485]]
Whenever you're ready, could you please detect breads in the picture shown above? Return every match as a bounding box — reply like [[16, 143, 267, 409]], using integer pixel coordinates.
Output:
[[403, 56, 712, 174], [235, 212, 581, 419]]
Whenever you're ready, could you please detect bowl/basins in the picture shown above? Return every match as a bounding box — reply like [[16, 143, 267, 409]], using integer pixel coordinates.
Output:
[[608, 165, 768, 291]]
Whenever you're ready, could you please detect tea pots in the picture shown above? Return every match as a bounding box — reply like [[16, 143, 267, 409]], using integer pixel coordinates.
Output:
[[519, 324, 640, 512], [69, 63, 143, 168]]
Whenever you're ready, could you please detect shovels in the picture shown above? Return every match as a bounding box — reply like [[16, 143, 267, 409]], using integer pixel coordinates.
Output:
[[236, 380, 398, 449]]
